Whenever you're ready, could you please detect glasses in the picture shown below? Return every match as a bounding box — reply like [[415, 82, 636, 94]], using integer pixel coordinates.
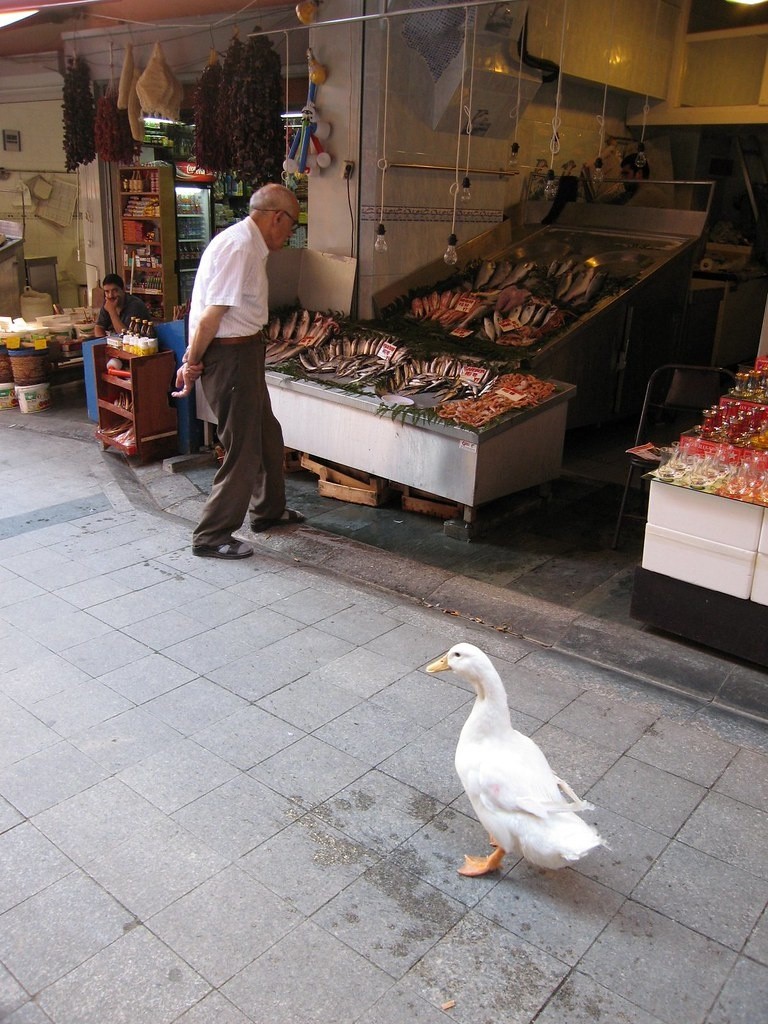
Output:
[[276, 209, 300, 231]]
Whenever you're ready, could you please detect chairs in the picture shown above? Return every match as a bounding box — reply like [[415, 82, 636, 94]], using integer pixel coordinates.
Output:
[[610, 363, 736, 549]]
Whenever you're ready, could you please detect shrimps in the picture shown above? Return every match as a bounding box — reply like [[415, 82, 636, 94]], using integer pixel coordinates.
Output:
[[435, 373, 557, 426]]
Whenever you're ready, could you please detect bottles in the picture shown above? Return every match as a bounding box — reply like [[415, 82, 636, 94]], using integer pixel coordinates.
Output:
[[155, 255, 160, 264], [178, 243, 201, 269], [181, 272, 196, 306], [295, 194, 307, 212], [215, 171, 264, 196], [121, 170, 151, 192], [174, 138, 198, 156], [120, 316, 158, 356], [126, 280, 130, 291], [178, 218, 202, 239], [177, 195, 201, 214]]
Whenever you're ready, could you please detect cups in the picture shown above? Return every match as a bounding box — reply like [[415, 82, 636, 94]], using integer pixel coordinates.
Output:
[[659, 365, 768, 506]]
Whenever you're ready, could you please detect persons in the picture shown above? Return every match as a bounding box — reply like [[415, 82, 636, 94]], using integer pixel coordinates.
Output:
[[607, 152, 675, 208], [172, 184, 305, 560], [93, 273, 150, 339]]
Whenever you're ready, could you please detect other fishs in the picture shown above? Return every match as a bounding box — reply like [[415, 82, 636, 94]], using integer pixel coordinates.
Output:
[[409, 259, 610, 347], [264, 308, 497, 404]]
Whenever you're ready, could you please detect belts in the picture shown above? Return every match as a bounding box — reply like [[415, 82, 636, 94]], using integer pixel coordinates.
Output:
[[215, 330, 264, 345]]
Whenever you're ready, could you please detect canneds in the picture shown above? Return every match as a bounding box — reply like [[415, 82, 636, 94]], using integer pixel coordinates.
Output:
[[176, 193, 202, 214]]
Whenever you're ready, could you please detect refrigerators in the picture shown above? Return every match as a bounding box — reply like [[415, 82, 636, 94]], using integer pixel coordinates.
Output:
[[174, 181, 215, 315]]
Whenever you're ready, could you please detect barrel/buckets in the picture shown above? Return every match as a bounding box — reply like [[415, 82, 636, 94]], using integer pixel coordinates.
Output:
[[54, 321, 96, 340], [0, 345, 54, 415]]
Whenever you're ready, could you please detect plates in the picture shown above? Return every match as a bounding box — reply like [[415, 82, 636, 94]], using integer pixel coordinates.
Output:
[[382, 394, 415, 406]]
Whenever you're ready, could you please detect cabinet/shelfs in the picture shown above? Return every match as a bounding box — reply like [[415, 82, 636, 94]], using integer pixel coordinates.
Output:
[[91, 344, 179, 469], [117, 165, 178, 323], [629, 355, 768, 668]]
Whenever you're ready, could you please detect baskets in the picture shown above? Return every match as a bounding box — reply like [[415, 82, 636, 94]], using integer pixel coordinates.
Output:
[[0, 354, 50, 386]]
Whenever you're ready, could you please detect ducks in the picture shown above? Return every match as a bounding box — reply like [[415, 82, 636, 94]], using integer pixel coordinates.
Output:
[[425, 642, 602, 878]]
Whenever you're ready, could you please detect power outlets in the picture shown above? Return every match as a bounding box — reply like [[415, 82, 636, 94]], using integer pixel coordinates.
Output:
[[341, 160, 354, 180]]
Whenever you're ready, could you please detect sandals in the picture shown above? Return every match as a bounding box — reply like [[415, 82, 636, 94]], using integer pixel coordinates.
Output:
[[192, 540, 253, 560], [250, 508, 305, 533]]
